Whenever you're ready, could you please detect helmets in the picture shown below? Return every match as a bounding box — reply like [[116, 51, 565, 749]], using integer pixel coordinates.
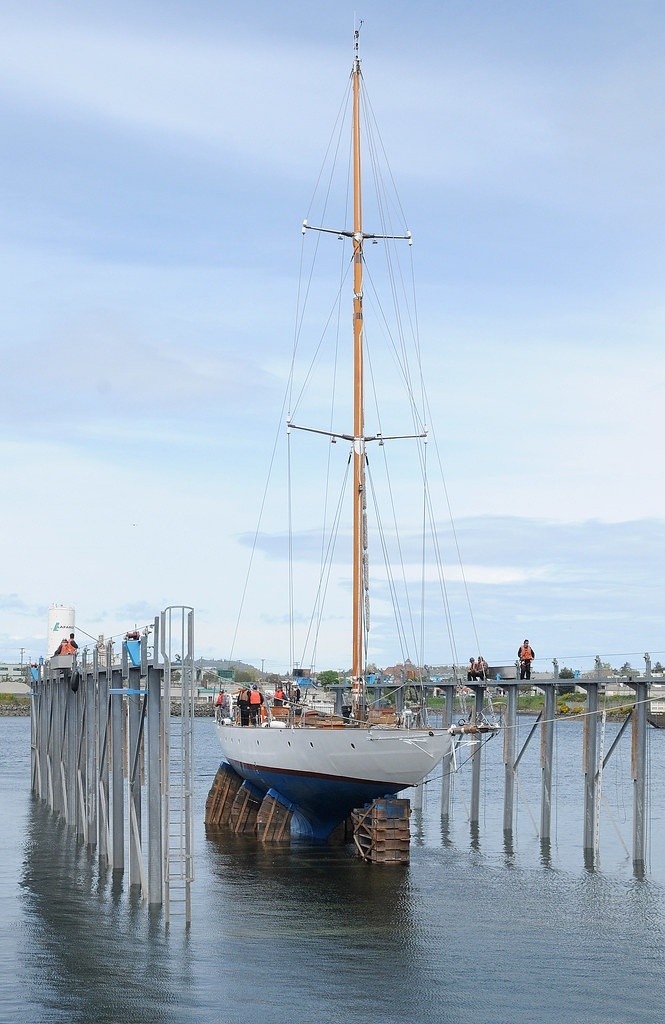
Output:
[[252, 685, 257, 688]]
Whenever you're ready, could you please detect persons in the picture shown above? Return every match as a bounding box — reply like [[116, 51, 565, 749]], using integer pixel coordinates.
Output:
[[238, 687, 251, 727], [275, 686, 287, 706], [215, 689, 227, 709], [474, 656, 488, 680], [467, 657, 478, 682], [67, 633, 78, 655], [519, 639, 535, 680], [49, 638, 68, 658], [249, 685, 264, 727]]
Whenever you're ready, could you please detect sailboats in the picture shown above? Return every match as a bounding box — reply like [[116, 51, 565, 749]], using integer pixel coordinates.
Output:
[[213, 22, 504, 845]]
